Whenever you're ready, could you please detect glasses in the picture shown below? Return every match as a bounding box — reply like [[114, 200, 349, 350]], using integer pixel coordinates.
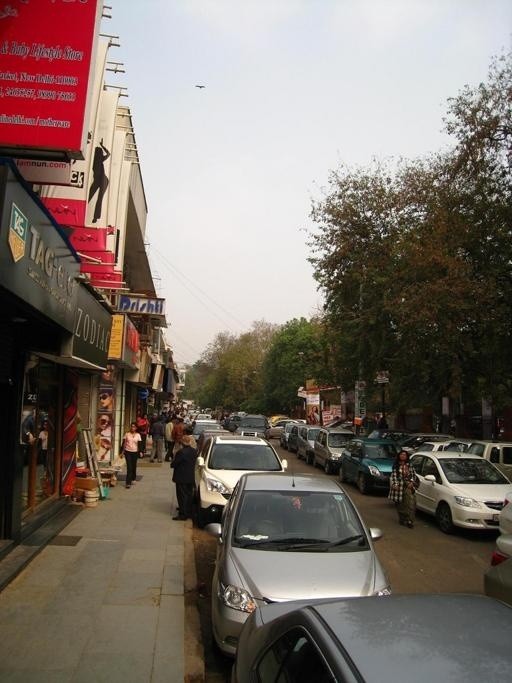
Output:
[[104, 369, 112, 372], [99, 394, 108, 400], [101, 419, 111, 425]]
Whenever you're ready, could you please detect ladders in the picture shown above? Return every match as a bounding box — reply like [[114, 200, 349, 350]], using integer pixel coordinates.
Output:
[[82, 428, 105, 498]]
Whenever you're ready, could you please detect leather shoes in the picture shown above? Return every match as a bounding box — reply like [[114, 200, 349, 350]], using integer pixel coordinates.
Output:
[[407, 523, 413, 528], [172, 516, 186, 520]]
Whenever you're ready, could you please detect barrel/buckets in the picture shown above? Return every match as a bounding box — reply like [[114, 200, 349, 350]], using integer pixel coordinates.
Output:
[[84, 490, 99, 507]]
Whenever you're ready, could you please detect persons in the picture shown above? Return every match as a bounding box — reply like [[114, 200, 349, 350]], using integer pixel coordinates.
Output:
[[97, 389, 112, 411], [21, 409, 52, 450], [96, 413, 113, 435], [306, 406, 320, 424], [387, 450, 420, 528], [119, 407, 231, 521], [99, 364, 116, 384], [94, 437, 108, 462]]
